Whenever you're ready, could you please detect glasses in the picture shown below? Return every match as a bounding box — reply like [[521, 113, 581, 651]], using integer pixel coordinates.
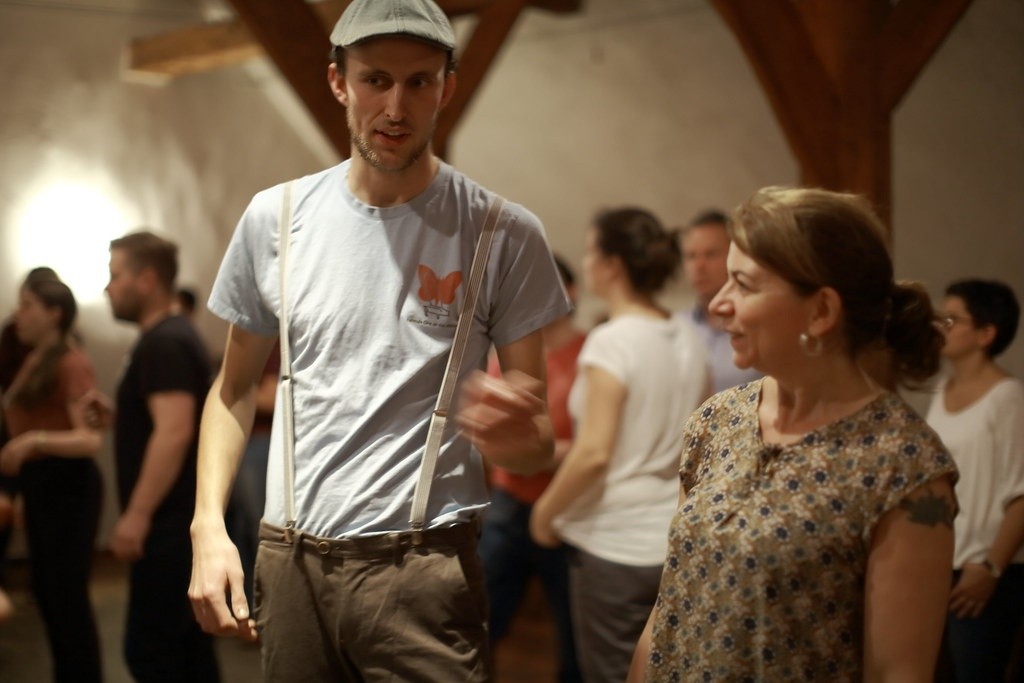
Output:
[[942, 316, 974, 330]]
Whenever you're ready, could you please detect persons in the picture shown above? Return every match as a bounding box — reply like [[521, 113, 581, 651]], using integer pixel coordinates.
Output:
[[914, 279, 1024, 683], [479, 251, 586, 683], [80, 232, 225, 683], [530, 205, 713, 683], [673, 209, 764, 392], [177, 286, 279, 652], [628, 187, 960, 683], [188, 0, 573, 683], [0, 267, 118, 683]]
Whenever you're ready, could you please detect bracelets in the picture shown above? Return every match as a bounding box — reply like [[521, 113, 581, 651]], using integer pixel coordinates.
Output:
[[969, 556, 1002, 578]]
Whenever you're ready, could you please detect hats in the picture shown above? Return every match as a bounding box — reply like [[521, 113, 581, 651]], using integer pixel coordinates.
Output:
[[330, 0, 456, 52]]
